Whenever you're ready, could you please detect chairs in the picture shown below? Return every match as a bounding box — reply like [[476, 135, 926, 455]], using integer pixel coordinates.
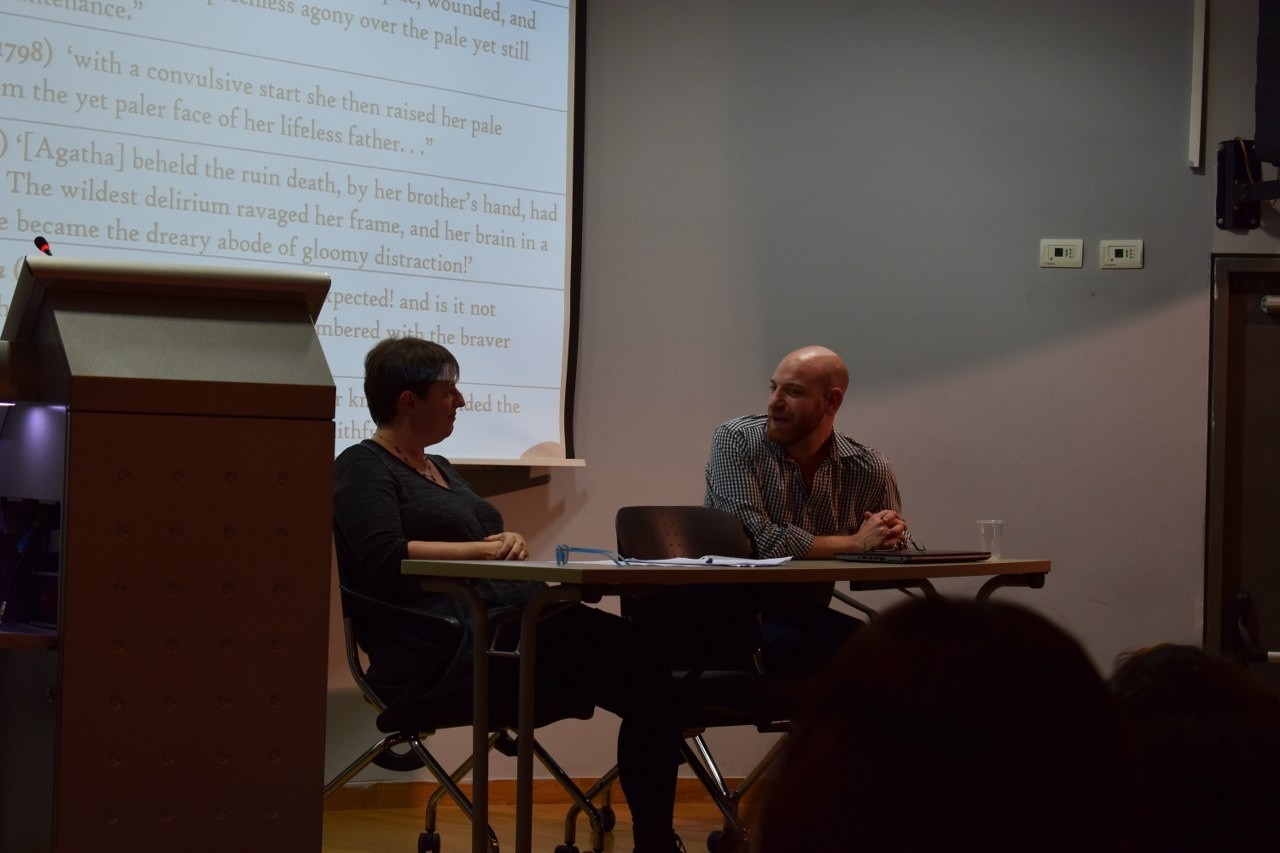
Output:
[[705, 588, 880, 853], [554, 506, 801, 853], [324, 517, 604, 853]]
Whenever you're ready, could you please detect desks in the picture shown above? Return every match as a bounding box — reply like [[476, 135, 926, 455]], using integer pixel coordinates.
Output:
[[400, 548, 1052, 853]]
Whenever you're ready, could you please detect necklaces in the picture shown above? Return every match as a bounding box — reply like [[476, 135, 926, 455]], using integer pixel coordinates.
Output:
[[374, 433, 436, 482]]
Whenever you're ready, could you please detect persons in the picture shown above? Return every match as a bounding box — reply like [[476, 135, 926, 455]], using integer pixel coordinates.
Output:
[[704, 345, 911, 679], [738, 593, 1280, 853], [330, 337, 689, 853]]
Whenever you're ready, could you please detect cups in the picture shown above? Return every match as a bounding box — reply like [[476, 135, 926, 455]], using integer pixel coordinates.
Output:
[[976, 520, 1006, 559]]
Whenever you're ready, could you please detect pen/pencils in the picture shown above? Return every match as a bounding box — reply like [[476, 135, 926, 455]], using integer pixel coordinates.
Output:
[[706, 558, 713, 564]]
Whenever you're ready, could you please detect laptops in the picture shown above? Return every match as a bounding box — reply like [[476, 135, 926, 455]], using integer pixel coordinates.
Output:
[[835, 549, 992, 565]]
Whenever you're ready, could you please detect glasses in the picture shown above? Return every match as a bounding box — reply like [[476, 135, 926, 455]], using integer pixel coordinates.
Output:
[[556, 545, 631, 568]]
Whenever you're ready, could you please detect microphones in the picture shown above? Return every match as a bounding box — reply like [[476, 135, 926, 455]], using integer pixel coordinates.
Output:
[[34, 236, 53, 256]]
